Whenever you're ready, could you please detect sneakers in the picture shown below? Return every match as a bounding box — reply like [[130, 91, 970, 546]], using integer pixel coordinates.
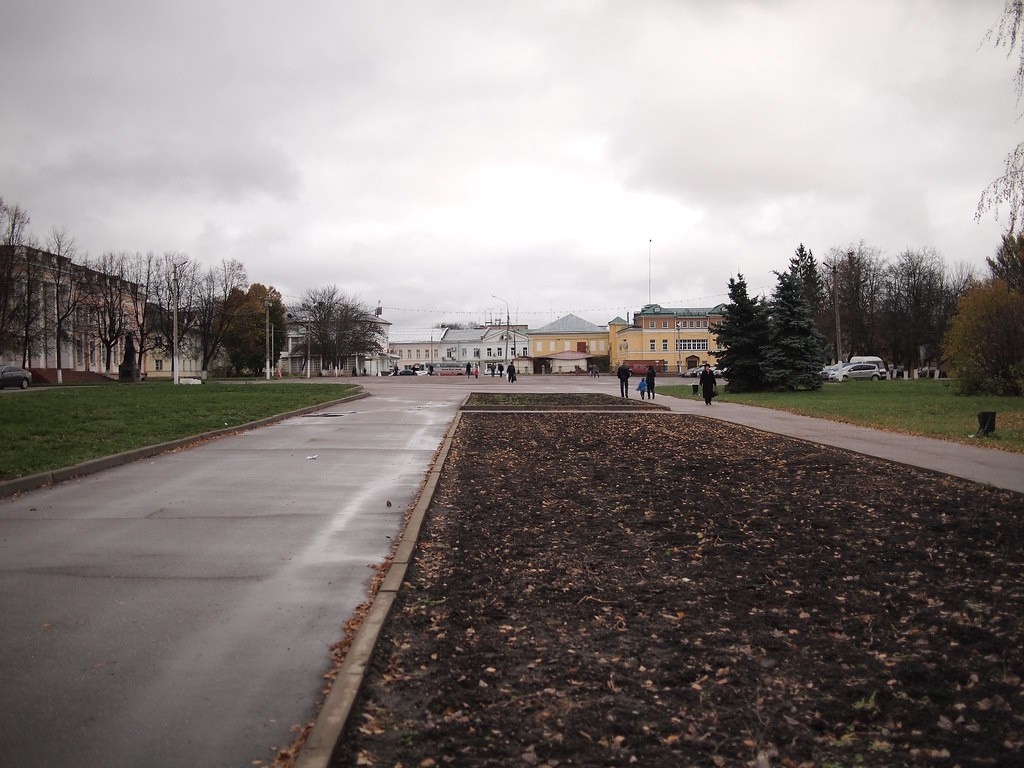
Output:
[[706, 402, 711, 405]]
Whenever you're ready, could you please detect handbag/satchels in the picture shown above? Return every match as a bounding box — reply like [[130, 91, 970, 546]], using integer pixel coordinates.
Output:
[[513, 375, 517, 381], [713, 391, 719, 396]]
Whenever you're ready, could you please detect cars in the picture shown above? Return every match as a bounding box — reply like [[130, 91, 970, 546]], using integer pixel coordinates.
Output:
[[395, 364, 506, 375], [0, 364, 33, 390], [682, 362, 729, 377], [815, 352, 890, 383]]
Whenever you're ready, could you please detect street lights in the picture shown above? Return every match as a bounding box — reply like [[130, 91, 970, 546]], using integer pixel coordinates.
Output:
[[173, 262, 190, 384], [823, 261, 843, 384]]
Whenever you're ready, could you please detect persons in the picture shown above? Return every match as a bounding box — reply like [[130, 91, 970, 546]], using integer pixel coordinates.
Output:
[[466, 362, 471, 375], [363, 368, 366, 375], [636, 378, 647, 400], [429, 364, 433, 375], [588, 363, 593, 376], [507, 362, 515, 383], [700, 364, 717, 405], [395, 365, 398, 376], [617, 362, 630, 398], [474, 369, 479, 378], [491, 365, 495, 377], [593, 363, 600, 378], [411, 365, 416, 375], [646, 366, 656, 399], [498, 364, 504, 377]]
